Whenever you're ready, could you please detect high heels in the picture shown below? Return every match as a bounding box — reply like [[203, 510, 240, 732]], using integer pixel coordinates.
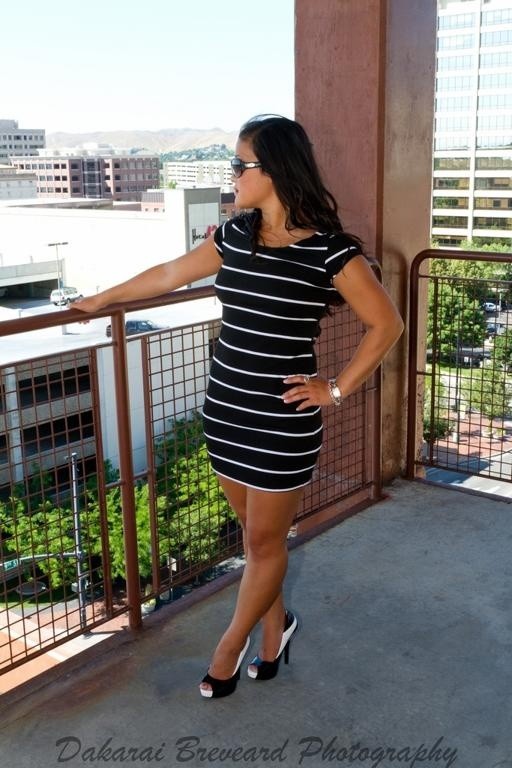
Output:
[[246, 607, 300, 684], [199, 632, 251, 699]]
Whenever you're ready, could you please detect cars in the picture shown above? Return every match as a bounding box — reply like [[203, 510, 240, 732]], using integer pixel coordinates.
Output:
[[50, 287, 84, 306], [481, 302, 502, 312], [485, 321, 507, 341]]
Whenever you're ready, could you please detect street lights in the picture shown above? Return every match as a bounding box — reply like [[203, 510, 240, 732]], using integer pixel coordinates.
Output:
[[494, 288, 508, 321], [49, 242, 69, 335]]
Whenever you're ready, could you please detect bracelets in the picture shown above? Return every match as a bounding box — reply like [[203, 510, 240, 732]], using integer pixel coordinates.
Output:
[[326, 377, 344, 407]]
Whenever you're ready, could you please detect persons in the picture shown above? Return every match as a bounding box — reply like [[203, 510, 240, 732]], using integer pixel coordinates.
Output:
[[66, 114, 406, 700]]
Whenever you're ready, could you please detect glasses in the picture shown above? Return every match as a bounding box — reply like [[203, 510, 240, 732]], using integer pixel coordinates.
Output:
[[230, 158, 262, 178]]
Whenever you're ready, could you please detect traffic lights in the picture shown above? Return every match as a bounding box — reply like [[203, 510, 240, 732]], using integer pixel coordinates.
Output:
[[84, 582, 94, 595]]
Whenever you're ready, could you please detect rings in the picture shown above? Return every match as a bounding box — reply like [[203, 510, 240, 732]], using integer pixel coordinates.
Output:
[[303, 374, 309, 385]]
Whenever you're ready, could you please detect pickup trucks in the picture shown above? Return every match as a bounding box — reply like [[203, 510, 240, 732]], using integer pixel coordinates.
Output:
[[106, 320, 170, 338]]
